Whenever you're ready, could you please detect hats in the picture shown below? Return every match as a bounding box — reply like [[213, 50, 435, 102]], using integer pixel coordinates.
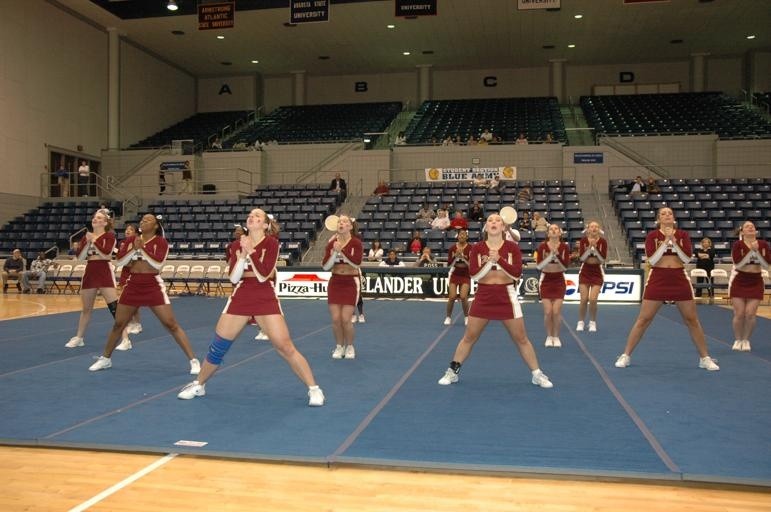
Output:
[[13, 249, 20, 253]]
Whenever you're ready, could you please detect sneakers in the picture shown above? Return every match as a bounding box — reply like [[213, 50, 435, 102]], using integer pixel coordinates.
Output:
[[89, 355, 113, 371], [552, 336, 561, 348], [64, 337, 85, 349], [131, 323, 143, 334], [127, 323, 134, 333], [307, 389, 324, 406], [615, 354, 630, 369], [189, 359, 201, 375], [262, 334, 269, 340], [330, 344, 345, 359], [345, 345, 355, 359], [255, 331, 263, 339], [177, 380, 205, 399], [531, 369, 554, 388], [351, 315, 357, 324], [732, 340, 741, 352], [358, 314, 365, 322], [438, 367, 460, 386], [698, 356, 720, 371], [444, 316, 451, 325], [576, 321, 585, 331], [589, 321, 596, 333], [545, 336, 553, 347], [115, 340, 132, 351], [464, 317, 469, 324], [742, 340, 751, 352]]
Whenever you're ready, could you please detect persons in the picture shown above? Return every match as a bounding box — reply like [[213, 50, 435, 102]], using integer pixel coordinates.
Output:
[[232, 139, 280, 152], [321, 216, 363, 359], [470, 200, 485, 221], [434, 203, 451, 220], [645, 176, 659, 193], [427, 128, 505, 147], [474, 174, 485, 186], [544, 135, 553, 143], [226, 227, 258, 325], [535, 224, 570, 348], [438, 214, 554, 389], [1, 249, 26, 294], [117, 224, 143, 335], [344, 218, 365, 323], [23, 253, 47, 295], [489, 176, 500, 189], [728, 221, 770, 352], [501, 219, 520, 246], [430, 209, 450, 230], [575, 221, 608, 332], [379, 250, 405, 267], [372, 181, 390, 197], [78, 160, 90, 197], [626, 177, 648, 193], [395, 130, 407, 145], [58, 165, 70, 197], [69, 241, 79, 255], [415, 247, 438, 268], [256, 266, 277, 341], [451, 210, 469, 230], [212, 138, 222, 151], [569, 240, 582, 266], [88, 214, 201, 375], [416, 202, 434, 224], [407, 231, 426, 254], [444, 228, 474, 326], [518, 187, 530, 204], [518, 212, 530, 231], [65, 209, 132, 351], [367, 239, 383, 263], [614, 208, 719, 371], [329, 173, 348, 204], [531, 212, 550, 232], [515, 133, 527, 145], [177, 209, 325, 407], [694, 238, 716, 304]]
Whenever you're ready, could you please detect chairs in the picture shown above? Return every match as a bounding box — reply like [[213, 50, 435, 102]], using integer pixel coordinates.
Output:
[[159, 265, 176, 295], [0, 199, 121, 258], [45, 260, 61, 294], [761, 267, 771, 304], [215, 266, 229, 296], [708, 268, 728, 306], [3, 259, 27, 284], [354, 181, 587, 265], [131, 108, 249, 153], [580, 89, 770, 141], [394, 95, 571, 149], [222, 99, 403, 152], [168, 265, 192, 296], [51, 264, 75, 295], [609, 177, 770, 263], [64, 265, 86, 294], [688, 269, 710, 299], [114, 183, 348, 266], [196, 265, 225, 297], [182, 265, 207, 295]]
